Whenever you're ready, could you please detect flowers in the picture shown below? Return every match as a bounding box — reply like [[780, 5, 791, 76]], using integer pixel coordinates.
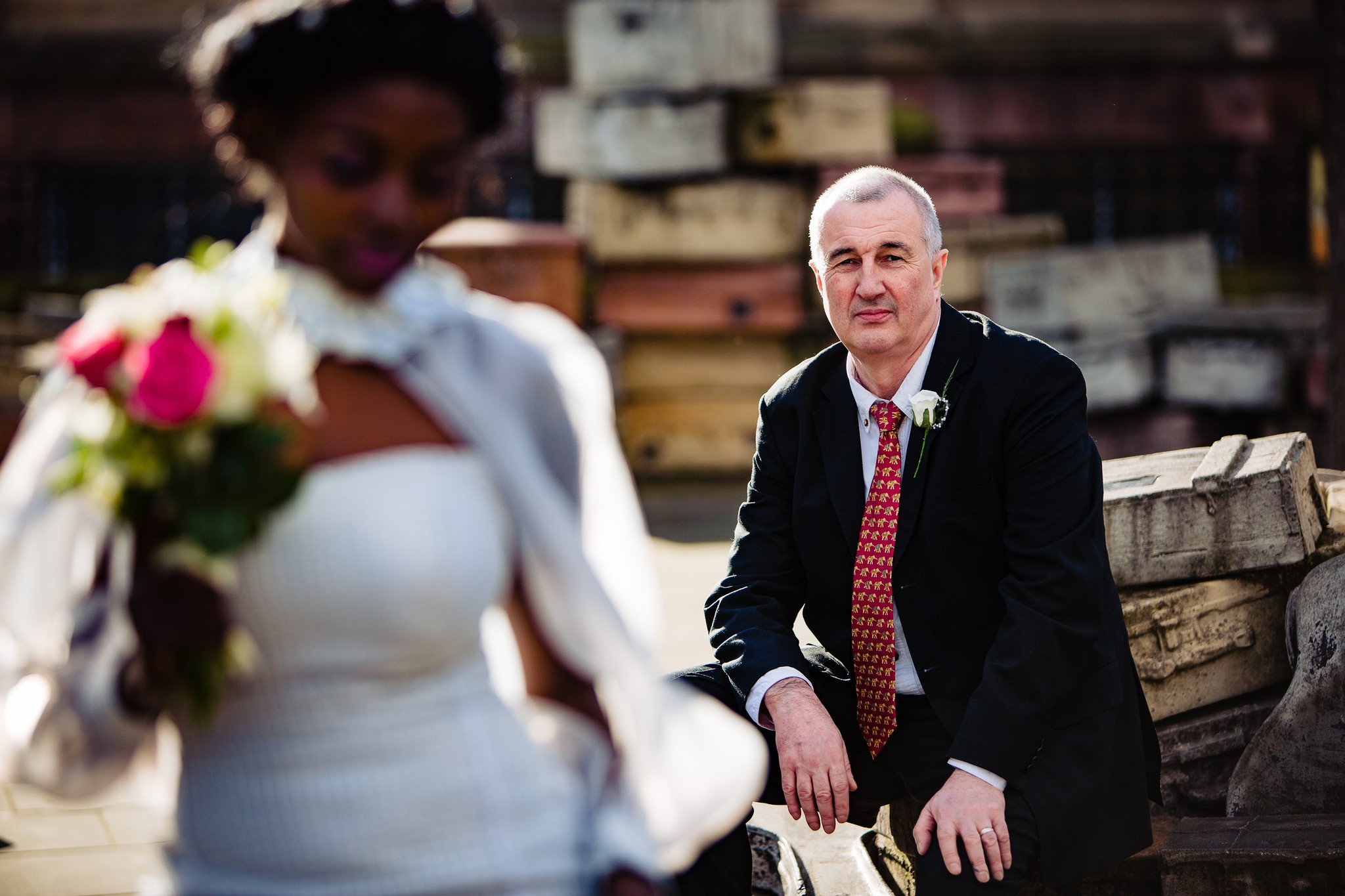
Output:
[[44, 231, 327, 742], [908, 358, 960, 479]]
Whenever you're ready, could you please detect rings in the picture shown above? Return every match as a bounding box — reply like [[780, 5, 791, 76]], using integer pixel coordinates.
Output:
[[980, 827, 994, 836]]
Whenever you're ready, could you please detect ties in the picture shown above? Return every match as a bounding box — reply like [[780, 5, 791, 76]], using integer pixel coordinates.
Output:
[[850, 401, 901, 761]]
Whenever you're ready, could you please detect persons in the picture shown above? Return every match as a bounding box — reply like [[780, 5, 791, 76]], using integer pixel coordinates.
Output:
[[0, 0, 772, 896], [657, 164, 1181, 896]]
